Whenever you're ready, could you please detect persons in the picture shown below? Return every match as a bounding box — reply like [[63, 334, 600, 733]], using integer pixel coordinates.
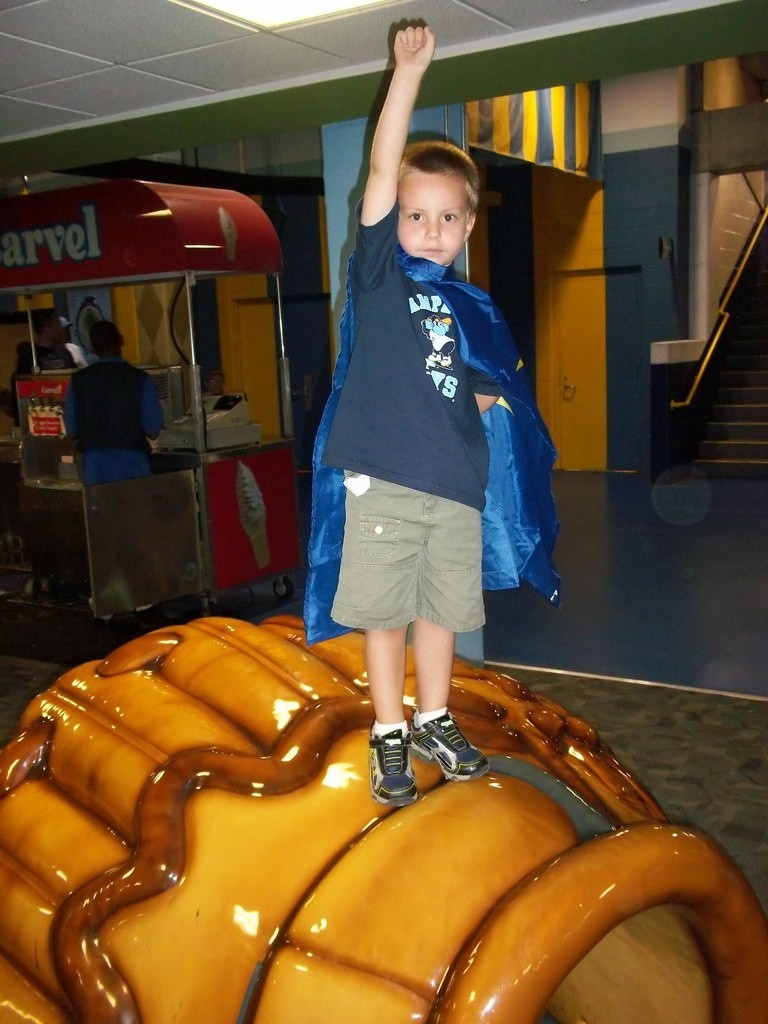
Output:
[[320, 27, 507, 807], [61, 319, 166, 652], [10, 309, 75, 426]]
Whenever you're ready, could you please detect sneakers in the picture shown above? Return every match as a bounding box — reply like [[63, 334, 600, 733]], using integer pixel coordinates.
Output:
[[411, 707, 490, 782], [369, 723, 418, 807]]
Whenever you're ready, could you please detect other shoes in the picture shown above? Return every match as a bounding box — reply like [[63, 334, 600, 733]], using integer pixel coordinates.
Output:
[[94, 618, 117, 632], [134, 604, 172, 626]]
